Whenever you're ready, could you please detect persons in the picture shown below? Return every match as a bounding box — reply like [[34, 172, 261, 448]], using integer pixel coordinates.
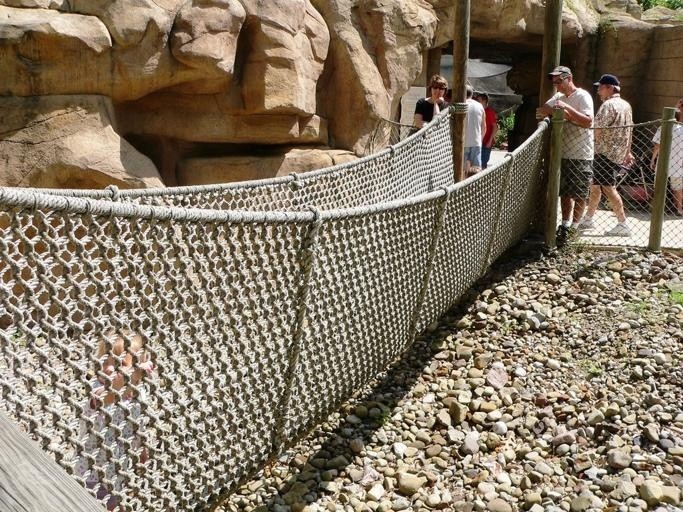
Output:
[[407, 74, 449, 136], [442, 85, 497, 178], [649, 98, 682, 216], [574, 73, 635, 237], [535, 65, 595, 248]]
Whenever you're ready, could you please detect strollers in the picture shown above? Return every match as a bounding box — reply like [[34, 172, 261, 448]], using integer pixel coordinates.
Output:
[[602, 146, 655, 212]]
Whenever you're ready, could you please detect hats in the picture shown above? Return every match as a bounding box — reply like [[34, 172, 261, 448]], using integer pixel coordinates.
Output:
[[549, 66, 571, 76], [594, 75, 619, 86]]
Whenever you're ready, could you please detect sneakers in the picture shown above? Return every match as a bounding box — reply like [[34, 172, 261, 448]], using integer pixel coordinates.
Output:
[[578, 220, 595, 230], [557, 225, 578, 246], [607, 223, 631, 237]]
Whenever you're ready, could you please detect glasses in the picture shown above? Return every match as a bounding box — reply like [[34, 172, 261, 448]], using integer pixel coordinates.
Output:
[[552, 76, 568, 85]]
